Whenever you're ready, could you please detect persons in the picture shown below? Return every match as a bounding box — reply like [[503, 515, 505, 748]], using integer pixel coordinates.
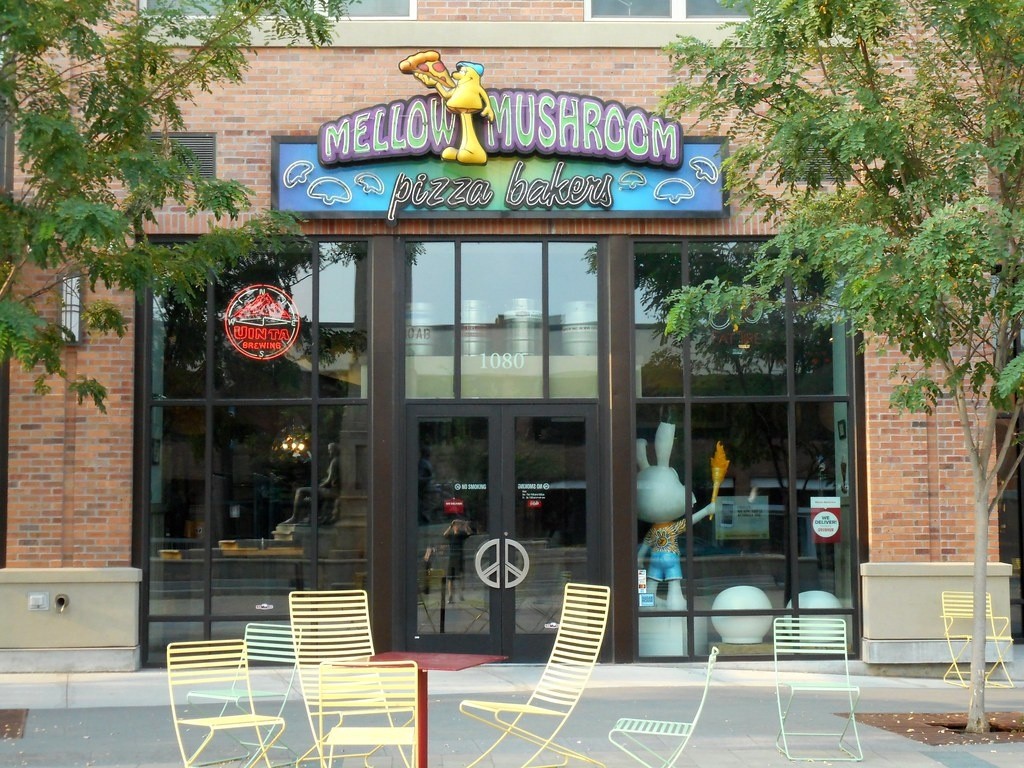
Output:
[[443, 520, 473, 604], [418, 443, 435, 526], [421, 547, 434, 594], [281, 443, 341, 524]]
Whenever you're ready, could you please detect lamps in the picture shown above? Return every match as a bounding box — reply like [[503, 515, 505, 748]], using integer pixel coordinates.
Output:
[[57, 270, 83, 349]]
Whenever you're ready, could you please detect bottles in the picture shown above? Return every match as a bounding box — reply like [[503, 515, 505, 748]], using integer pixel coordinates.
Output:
[[816, 455, 826, 481]]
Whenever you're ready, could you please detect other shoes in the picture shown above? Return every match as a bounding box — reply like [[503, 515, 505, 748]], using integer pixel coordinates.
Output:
[[458, 595, 464, 601], [446, 596, 453, 604]]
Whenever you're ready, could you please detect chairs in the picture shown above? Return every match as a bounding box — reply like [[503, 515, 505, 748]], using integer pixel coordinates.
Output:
[[460, 582, 611, 768], [773, 617, 864, 761], [166, 591, 418, 768], [415, 564, 569, 634], [940, 590, 1014, 689], [607, 645, 719, 768]]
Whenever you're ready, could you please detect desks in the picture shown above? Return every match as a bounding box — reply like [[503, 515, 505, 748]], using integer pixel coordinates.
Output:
[[333, 651, 507, 768], [416, 573, 463, 634]]
